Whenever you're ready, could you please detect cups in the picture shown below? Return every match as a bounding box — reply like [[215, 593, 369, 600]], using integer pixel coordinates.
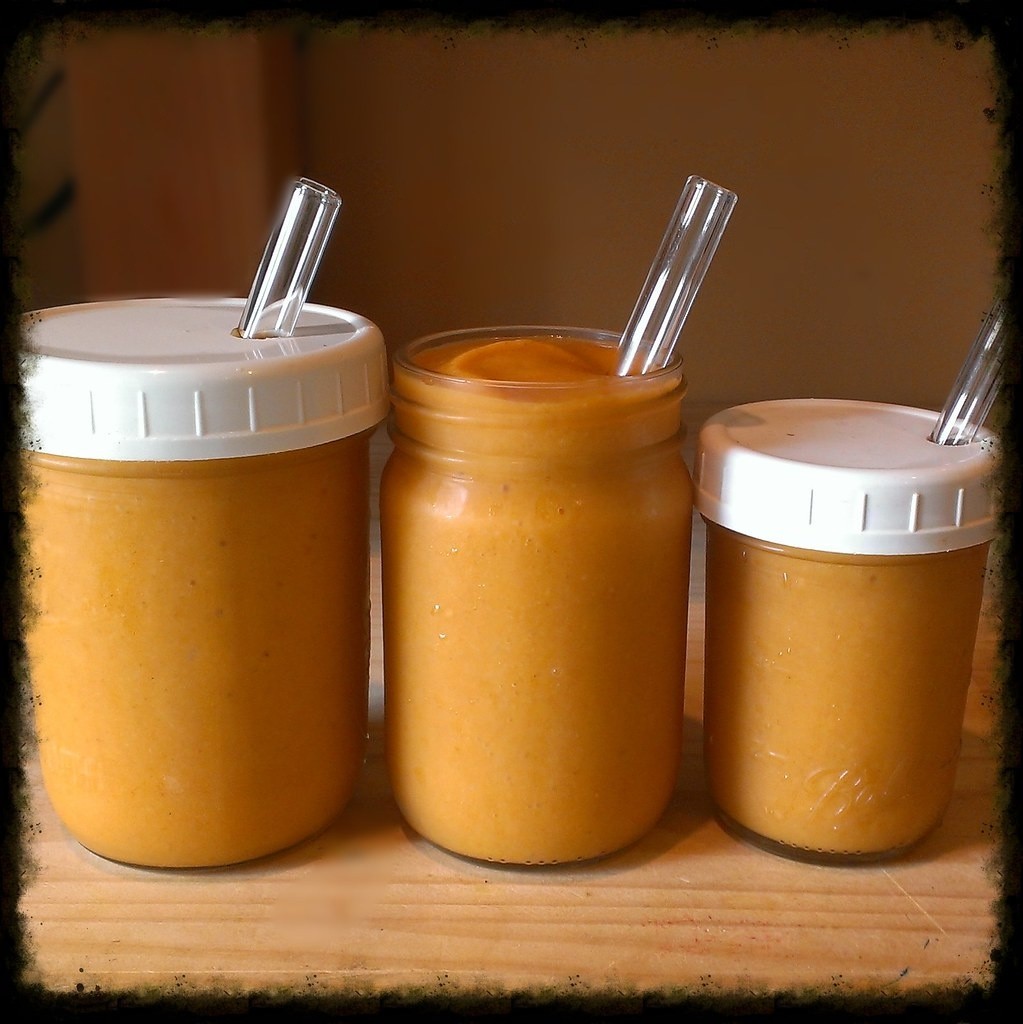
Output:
[[377, 324, 690, 865], [691, 399, 1004, 868], [21, 299, 389, 868]]
